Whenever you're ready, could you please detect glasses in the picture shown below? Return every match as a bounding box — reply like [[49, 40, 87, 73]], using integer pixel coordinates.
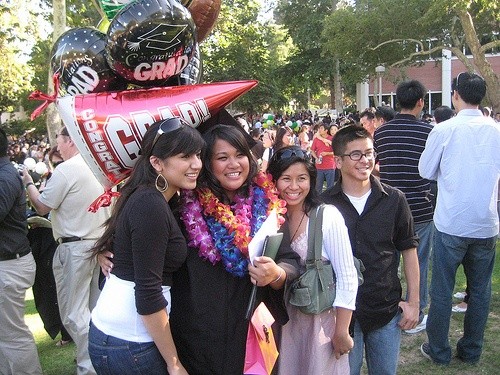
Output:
[[376, 106, 385, 116], [273, 149, 310, 167], [151, 116, 190, 150], [341, 150, 378, 161], [455, 72, 484, 87]]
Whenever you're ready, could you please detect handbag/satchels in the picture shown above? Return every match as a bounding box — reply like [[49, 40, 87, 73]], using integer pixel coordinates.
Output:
[[287, 205, 364, 314]]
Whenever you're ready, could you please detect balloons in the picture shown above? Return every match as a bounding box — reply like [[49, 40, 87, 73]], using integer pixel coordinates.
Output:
[[49, 0, 259, 190]]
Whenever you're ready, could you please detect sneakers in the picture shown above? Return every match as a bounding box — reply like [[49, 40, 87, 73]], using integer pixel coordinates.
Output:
[[405, 315, 428, 333]]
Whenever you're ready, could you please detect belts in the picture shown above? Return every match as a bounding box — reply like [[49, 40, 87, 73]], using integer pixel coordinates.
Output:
[[55, 236, 83, 245], [0, 246, 31, 262]]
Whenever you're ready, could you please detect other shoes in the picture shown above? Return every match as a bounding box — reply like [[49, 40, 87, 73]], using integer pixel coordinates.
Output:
[[55, 338, 72, 346], [453, 291, 468, 300], [420, 343, 434, 360], [451, 304, 468, 312]]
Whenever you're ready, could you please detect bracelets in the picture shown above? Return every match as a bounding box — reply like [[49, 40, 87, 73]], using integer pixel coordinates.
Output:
[[270, 264, 282, 285], [26, 182, 35, 189]]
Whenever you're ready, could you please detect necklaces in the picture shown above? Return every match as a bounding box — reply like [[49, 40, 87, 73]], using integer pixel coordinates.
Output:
[[285, 203, 308, 245]]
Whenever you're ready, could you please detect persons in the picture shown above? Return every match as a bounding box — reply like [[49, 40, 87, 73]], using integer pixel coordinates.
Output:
[[234, 104, 499, 311], [24, 126, 120, 375], [96, 119, 306, 375], [0, 124, 45, 375], [319, 125, 419, 375], [267, 144, 358, 375], [0, 133, 72, 347], [417, 73, 499, 365], [372, 79, 436, 333], [86, 118, 203, 375]]
